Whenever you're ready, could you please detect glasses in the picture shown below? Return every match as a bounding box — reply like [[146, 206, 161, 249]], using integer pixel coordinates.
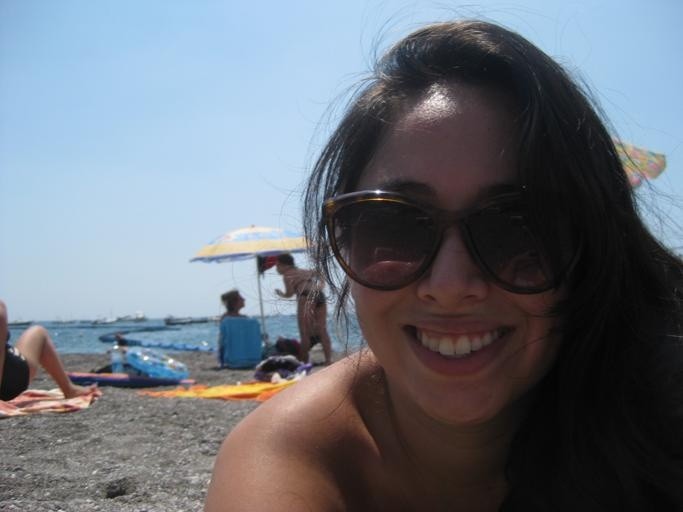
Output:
[[321, 183, 590, 294]]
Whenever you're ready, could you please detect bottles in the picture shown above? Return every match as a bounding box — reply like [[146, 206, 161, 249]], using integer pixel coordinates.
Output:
[[110, 340, 124, 373]]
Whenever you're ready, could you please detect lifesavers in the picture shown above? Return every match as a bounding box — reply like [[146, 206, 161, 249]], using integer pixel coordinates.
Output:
[[127, 346, 191, 380], [254, 355, 308, 380]]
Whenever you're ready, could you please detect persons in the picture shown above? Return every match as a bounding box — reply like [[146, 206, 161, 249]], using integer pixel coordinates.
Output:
[[0, 298, 98, 402], [212, 253, 333, 370], [200, 21, 683, 512], [106, 333, 129, 373]]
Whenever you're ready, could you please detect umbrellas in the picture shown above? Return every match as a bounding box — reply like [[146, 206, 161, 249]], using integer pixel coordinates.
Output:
[[189, 223, 319, 344]]
